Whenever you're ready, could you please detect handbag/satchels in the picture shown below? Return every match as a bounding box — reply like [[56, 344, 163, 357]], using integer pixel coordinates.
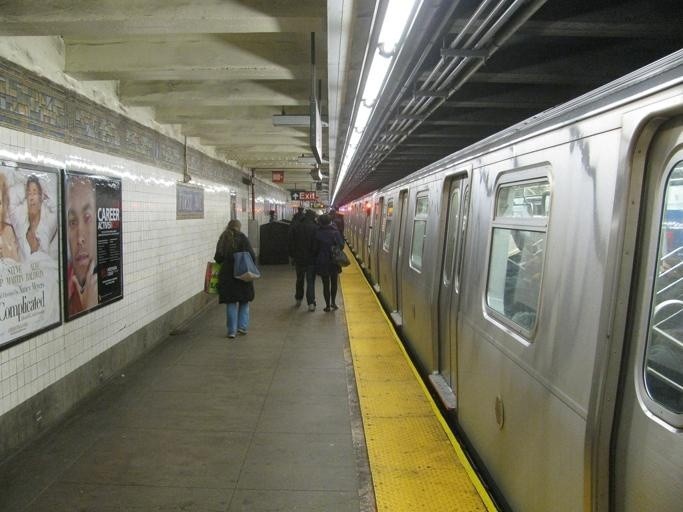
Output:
[[232, 251, 261, 282], [330, 244, 350, 267], [203, 262, 222, 294]]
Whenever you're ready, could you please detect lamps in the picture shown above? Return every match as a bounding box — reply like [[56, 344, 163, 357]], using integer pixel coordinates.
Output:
[[331, 0, 423, 206]]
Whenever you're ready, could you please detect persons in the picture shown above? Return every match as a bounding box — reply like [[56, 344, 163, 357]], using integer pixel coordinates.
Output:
[[1, 173, 22, 264], [65, 177, 99, 316], [289, 206, 345, 309], [15, 173, 56, 254], [214, 219, 256, 337]]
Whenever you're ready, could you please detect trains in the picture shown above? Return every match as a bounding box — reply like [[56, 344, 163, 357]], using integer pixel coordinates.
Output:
[[336, 47, 683, 511]]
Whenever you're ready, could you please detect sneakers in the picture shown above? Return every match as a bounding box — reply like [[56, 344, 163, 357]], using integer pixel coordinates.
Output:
[[237, 328, 248, 335], [296, 298, 338, 311], [227, 333, 236, 338]]
